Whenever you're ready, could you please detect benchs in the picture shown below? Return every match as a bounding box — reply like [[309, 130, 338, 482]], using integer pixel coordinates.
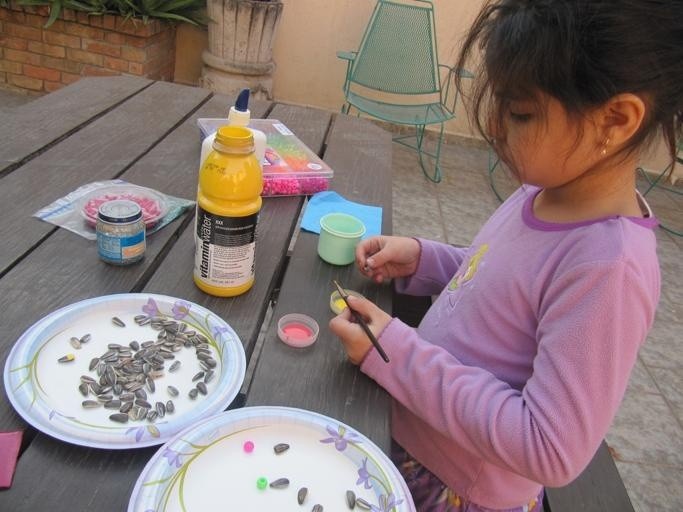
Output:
[[541, 439, 635, 512]]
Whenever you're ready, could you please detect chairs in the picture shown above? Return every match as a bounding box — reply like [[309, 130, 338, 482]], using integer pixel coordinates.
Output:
[[337, 0, 474, 186]]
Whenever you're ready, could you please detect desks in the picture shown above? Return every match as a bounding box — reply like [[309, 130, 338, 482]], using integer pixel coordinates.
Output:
[[0, 74, 391, 510]]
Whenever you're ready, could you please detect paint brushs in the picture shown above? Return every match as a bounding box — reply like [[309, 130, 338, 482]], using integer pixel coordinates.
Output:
[[332, 279, 390, 363]]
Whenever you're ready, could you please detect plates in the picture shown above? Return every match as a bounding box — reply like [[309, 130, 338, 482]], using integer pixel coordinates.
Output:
[[125, 404, 416, 512], [1, 291, 246, 451]]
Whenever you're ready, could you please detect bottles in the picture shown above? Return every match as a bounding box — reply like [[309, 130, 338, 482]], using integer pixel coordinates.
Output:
[[192, 87, 268, 296]]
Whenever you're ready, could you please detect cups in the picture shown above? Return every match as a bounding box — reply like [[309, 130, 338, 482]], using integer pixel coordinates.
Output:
[[318, 213, 367, 267]]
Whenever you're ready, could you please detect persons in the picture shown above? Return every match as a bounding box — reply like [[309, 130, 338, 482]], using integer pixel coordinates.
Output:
[[326, 0, 681, 512]]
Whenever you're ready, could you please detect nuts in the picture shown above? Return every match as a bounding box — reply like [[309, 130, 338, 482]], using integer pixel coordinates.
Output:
[[270, 477, 290, 490], [57, 313, 219, 426], [313, 503, 323, 512], [273, 442, 291, 454], [296, 486, 309, 505], [345, 489, 374, 510]]
